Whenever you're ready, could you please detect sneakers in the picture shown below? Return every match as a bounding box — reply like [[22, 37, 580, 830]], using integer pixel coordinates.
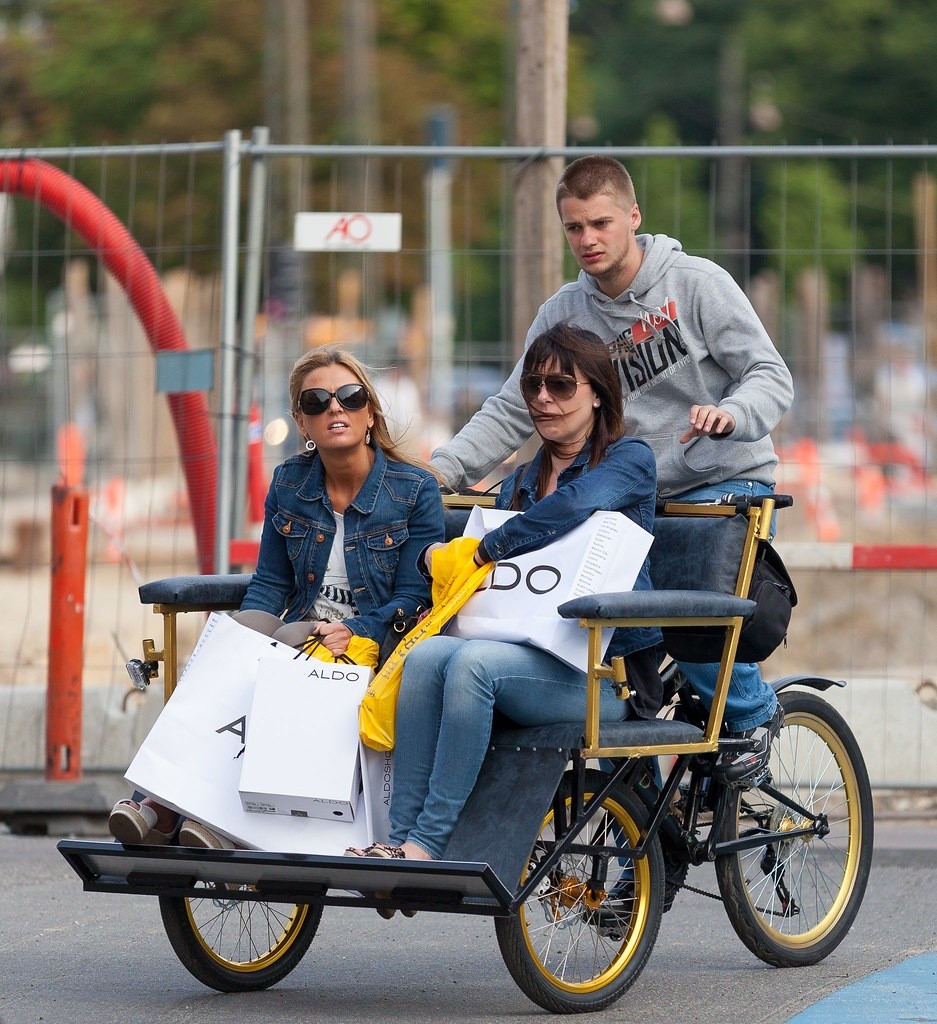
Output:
[[715, 701, 785, 783], [578, 879, 676, 924]]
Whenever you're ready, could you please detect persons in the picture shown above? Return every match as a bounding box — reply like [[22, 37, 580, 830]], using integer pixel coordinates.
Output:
[[109, 347, 446, 892], [425, 155, 795, 926], [344, 321, 663, 917]]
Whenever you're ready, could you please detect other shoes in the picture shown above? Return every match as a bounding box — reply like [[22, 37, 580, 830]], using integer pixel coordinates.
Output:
[[344, 841, 398, 919], [367, 843, 419, 919]]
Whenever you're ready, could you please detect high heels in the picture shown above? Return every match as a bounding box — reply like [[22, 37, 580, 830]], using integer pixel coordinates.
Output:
[[108, 798, 179, 845], [178, 818, 242, 889]]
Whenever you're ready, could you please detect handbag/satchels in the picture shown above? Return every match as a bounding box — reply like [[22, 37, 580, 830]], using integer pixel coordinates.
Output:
[[238, 635, 377, 822], [124, 609, 395, 856], [659, 538, 798, 663], [443, 504, 655, 674]]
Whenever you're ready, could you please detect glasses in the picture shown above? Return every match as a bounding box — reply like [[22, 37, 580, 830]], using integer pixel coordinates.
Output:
[[294, 384, 369, 416], [521, 372, 591, 400]]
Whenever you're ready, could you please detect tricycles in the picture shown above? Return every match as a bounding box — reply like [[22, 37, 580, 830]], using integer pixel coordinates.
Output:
[[57, 492, 876, 1018]]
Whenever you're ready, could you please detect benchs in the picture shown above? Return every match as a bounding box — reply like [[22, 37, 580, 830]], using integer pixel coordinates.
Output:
[[136, 492, 796, 759]]
